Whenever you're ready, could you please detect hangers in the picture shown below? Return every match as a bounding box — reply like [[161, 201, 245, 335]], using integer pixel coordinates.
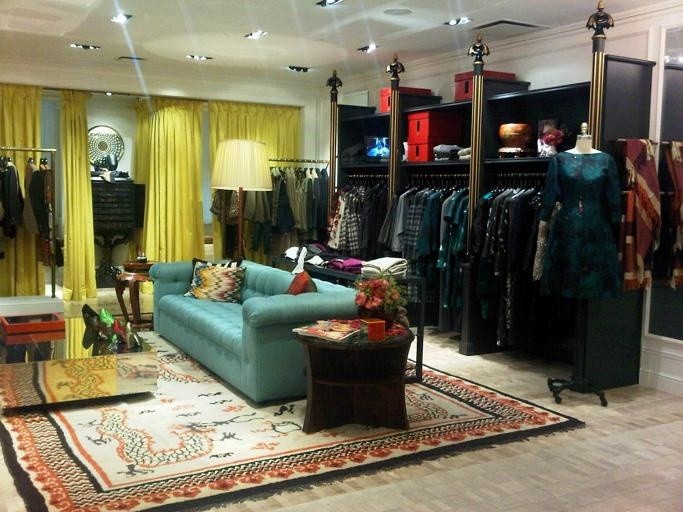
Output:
[[0, 146, 53, 163], [343, 172, 549, 192], [268, 156, 327, 172]]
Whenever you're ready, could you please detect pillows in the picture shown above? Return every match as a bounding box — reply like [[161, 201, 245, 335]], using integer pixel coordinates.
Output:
[[189, 257, 243, 288], [287, 271, 317, 295], [183, 262, 246, 304]]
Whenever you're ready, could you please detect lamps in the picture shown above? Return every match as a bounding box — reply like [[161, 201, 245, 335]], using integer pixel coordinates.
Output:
[[208, 138, 273, 258]]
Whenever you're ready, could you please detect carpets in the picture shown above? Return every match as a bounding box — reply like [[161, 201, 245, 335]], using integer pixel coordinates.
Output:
[[0, 317, 589, 512], [0, 350, 155, 412]]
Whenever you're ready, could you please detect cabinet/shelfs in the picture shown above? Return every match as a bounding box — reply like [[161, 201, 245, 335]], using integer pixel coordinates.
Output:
[[465, 1, 657, 396], [388, 32, 477, 358], [88, 178, 146, 288], [326, 52, 403, 225]]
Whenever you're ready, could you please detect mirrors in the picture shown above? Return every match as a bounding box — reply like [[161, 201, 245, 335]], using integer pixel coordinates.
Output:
[[643, 22, 681, 347]]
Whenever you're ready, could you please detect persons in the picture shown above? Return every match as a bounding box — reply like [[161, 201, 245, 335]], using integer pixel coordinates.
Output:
[[532, 134, 625, 301]]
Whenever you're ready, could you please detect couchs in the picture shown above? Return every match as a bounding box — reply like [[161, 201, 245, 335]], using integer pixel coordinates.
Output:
[[147, 257, 362, 407]]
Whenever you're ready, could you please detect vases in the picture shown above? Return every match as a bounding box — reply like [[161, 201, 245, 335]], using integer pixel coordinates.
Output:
[[366, 317, 386, 341]]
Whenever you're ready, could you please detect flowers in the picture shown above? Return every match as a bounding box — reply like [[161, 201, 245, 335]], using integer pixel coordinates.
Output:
[[353, 274, 408, 323]]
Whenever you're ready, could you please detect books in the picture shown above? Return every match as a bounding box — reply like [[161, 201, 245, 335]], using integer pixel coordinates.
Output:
[[292, 324, 360, 343]]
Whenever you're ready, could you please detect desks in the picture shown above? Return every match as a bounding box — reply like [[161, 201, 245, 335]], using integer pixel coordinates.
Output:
[[292, 316, 416, 434], [114, 270, 153, 331], [270, 254, 425, 385]]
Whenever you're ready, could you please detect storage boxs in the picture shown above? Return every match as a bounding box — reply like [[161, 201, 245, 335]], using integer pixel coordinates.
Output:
[[407, 138, 443, 163], [407, 110, 446, 139], [452, 68, 516, 101], [378, 85, 431, 114]]
